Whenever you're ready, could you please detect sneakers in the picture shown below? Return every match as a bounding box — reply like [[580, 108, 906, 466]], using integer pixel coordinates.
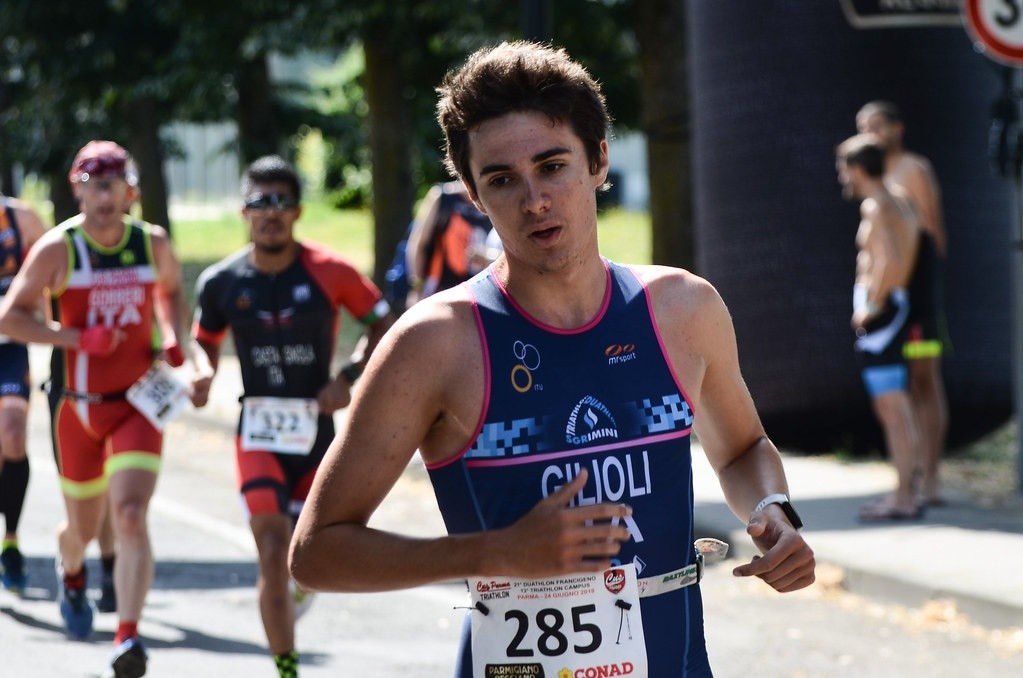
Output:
[[0, 546, 28, 592], [54, 552, 93, 639], [108, 634, 148, 678]]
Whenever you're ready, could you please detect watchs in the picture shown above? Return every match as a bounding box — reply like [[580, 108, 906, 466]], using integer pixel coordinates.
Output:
[[754, 493, 805, 532]]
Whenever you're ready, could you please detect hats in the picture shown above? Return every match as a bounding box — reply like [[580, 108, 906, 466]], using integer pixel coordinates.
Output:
[[67, 139, 130, 185]]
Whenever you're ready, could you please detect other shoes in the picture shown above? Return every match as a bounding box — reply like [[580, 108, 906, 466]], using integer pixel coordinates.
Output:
[[94, 573, 116, 614], [915, 484, 945, 509], [289, 578, 316, 622], [857, 495, 928, 525]]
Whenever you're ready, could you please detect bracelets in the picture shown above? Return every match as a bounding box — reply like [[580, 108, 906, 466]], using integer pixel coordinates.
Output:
[[340, 362, 361, 383]]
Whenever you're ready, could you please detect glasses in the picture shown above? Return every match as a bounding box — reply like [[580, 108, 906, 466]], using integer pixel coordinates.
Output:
[[243, 192, 299, 212]]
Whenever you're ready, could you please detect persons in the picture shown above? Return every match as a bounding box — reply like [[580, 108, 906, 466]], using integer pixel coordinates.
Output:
[[287, 41, 816, 678], [0, 141, 503, 678], [839, 133, 925, 526], [852, 103, 948, 507]]
